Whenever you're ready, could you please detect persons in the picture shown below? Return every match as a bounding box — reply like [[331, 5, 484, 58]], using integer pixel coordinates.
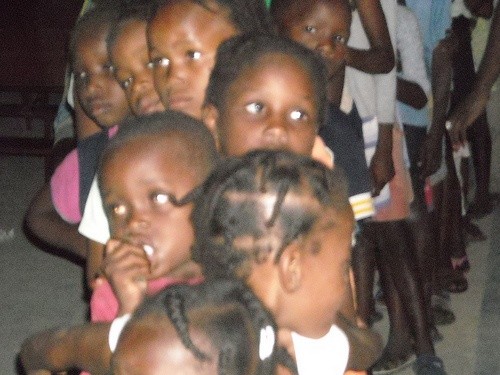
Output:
[[18, 149, 384, 375], [25, 0, 500, 375], [108, 279, 278, 375], [79, 109, 221, 375]]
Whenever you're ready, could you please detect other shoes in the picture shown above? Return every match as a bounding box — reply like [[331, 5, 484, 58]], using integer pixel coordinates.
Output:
[[365, 188, 500, 375]]
